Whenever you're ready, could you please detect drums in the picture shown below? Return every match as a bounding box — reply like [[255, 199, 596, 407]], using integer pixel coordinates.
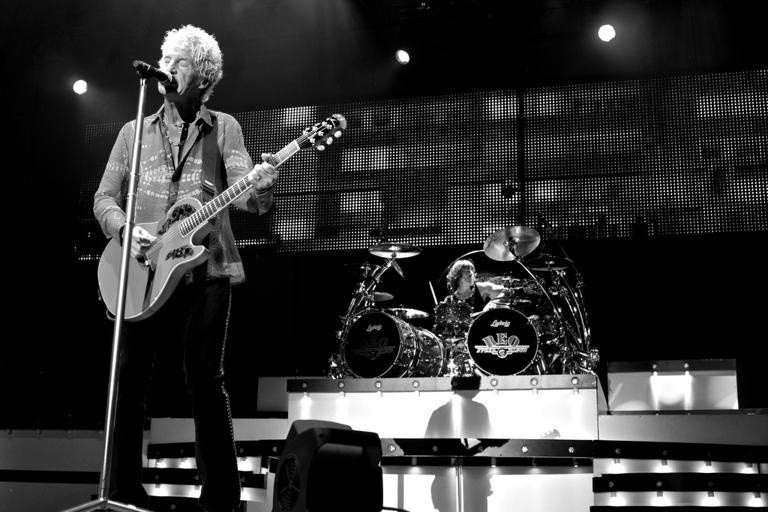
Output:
[[434, 303, 473, 325], [342, 311, 447, 378], [529, 315, 556, 340], [467, 309, 539, 376]]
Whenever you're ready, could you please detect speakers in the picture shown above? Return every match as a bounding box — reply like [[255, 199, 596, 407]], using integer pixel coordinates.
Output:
[[272, 419, 382, 512]]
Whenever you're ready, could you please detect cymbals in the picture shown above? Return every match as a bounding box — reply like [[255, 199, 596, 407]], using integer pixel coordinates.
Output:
[[492, 297, 531, 305], [363, 292, 393, 301], [358, 263, 378, 268], [527, 258, 570, 271], [386, 308, 430, 319], [368, 243, 421, 259], [483, 226, 541, 262]]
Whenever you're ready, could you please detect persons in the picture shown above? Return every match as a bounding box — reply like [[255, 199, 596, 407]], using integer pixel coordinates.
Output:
[[89, 22, 278, 512]]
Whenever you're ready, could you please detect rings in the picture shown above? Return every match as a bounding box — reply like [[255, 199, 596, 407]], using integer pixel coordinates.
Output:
[[254, 176, 262, 180]]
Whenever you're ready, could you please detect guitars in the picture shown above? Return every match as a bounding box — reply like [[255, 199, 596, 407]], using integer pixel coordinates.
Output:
[[97, 114, 347, 323]]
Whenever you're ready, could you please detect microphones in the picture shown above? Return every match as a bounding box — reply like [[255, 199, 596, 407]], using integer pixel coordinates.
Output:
[[133, 60, 174, 87]]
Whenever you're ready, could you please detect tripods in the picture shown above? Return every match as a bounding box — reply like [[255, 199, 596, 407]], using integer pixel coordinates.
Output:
[[62, 77, 152, 512]]
